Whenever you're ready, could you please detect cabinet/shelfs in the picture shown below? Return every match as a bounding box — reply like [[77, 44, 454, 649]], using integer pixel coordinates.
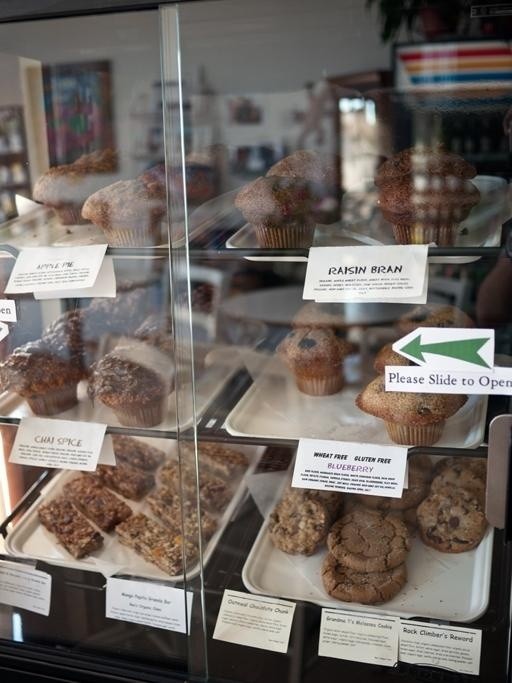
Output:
[[0, 1, 511, 683], [0, 102, 33, 220]]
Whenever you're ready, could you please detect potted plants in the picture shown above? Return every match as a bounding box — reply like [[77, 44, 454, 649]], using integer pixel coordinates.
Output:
[[360, 0, 512, 114]]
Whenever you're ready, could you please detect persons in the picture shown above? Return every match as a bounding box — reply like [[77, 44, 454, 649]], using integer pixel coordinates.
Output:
[[478, 108, 512, 329]]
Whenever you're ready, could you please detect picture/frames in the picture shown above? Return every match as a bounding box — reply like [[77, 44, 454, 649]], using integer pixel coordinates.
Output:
[[40, 57, 119, 176]]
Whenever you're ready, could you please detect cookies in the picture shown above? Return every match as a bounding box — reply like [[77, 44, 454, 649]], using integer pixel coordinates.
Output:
[[267, 453, 486, 605], [37, 434, 249, 578]]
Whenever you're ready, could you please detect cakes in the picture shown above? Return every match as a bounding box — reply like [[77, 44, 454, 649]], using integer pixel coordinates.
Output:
[[32, 145, 220, 247], [235, 152, 475, 250], [0, 289, 215, 426], [281, 300, 474, 445]]
